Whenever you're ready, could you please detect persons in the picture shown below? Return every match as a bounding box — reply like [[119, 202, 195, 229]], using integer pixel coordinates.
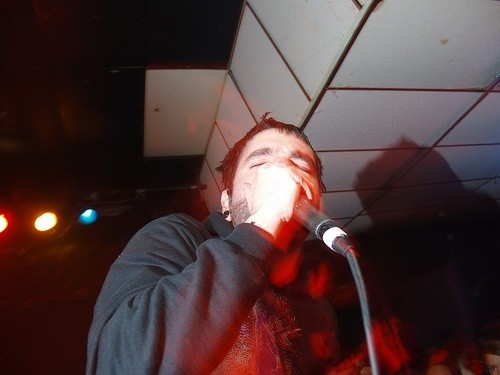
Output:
[[84, 110, 414, 375]]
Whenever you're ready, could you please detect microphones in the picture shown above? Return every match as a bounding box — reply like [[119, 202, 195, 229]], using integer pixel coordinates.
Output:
[[292, 195, 354, 256]]
